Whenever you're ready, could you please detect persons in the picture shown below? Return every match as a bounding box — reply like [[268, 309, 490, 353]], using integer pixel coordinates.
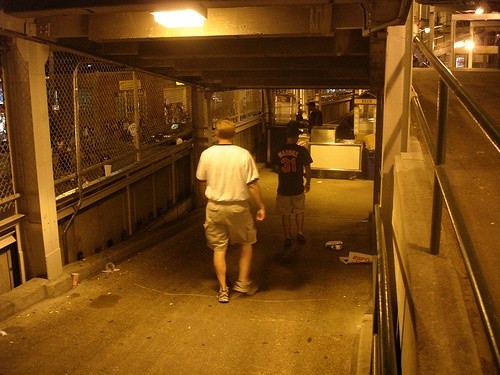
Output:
[[0, 141, 11, 178], [336, 117, 352, 140], [272, 126, 314, 247], [196, 118, 266, 303], [304, 102, 323, 132], [50, 119, 145, 194], [288, 114, 306, 128]]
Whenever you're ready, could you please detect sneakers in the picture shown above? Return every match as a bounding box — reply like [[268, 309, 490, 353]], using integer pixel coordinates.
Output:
[[232, 282, 259, 294], [297, 234, 304, 240], [218, 285, 229, 302], [285, 235, 292, 244]]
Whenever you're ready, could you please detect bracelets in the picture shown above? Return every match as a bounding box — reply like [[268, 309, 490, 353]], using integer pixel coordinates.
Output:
[[307, 183, 310, 185]]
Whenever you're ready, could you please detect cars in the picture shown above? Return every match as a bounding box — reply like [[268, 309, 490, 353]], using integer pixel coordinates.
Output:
[[152, 123, 186, 143]]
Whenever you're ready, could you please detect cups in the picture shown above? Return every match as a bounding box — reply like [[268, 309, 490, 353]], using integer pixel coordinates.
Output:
[[104, 165, 111, 177]]
